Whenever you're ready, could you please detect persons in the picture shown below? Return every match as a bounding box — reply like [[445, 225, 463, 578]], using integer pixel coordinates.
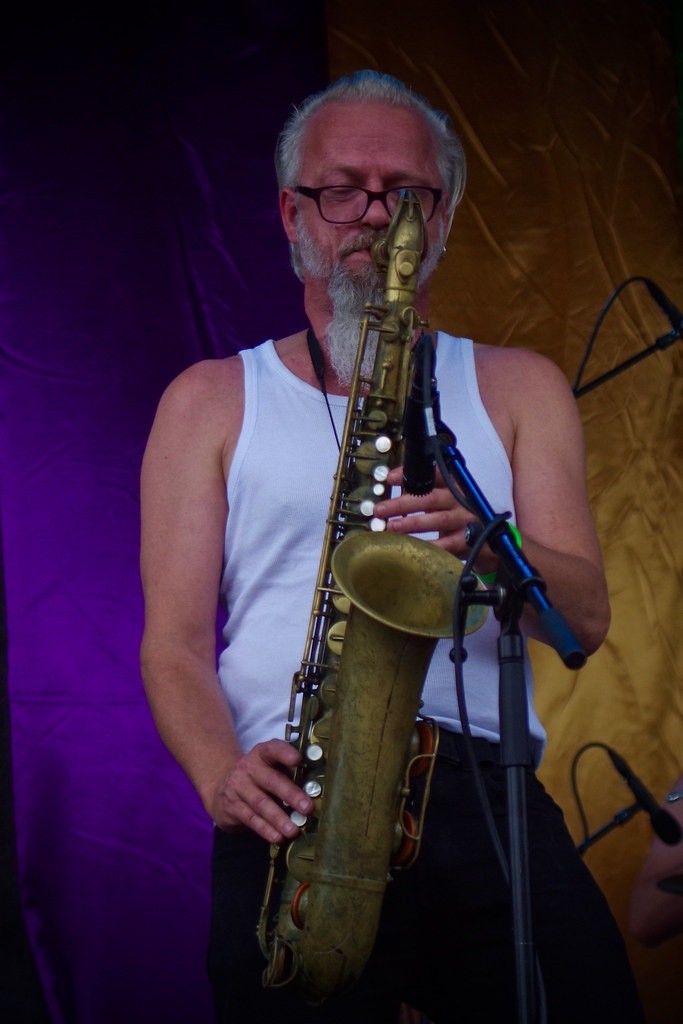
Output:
[[141, 69, 642, 1024], [630, 773, 683, 947]]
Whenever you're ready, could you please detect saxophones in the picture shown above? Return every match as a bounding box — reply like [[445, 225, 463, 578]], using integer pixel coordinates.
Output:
[[247, 186, 469, 1024]]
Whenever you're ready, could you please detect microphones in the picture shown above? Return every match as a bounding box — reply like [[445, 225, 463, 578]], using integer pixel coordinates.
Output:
[[402, 335, 436, 497], [606, 748, 683, 847], [646, 278, 683, 339]]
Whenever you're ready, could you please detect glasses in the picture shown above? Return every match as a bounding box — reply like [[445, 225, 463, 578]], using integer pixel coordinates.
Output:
[[295, 185, 443, 225]]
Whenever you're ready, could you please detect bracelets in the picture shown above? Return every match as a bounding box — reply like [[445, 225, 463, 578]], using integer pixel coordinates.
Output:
[[472, 522, 522, 591]]
